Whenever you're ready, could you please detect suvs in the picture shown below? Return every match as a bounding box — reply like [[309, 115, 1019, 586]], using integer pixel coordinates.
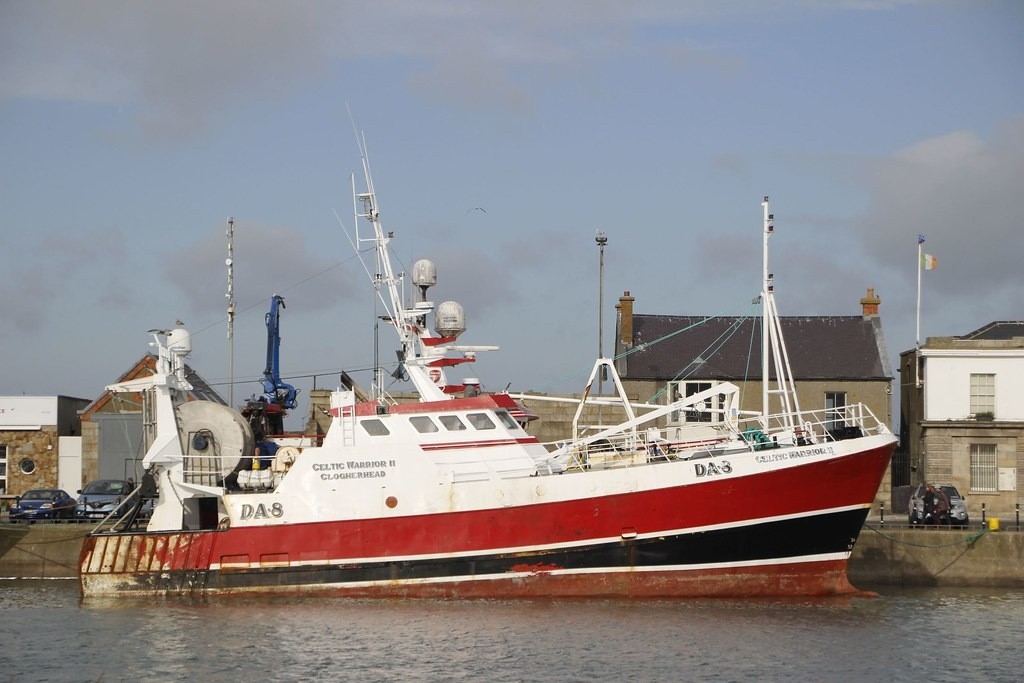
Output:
[[908, 483, 969, 528]]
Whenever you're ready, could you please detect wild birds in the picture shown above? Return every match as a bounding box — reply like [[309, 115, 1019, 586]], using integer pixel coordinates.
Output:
[[176, 318, 184, 327], [147, 328, 172, 337], [468, 207, 486, 214]]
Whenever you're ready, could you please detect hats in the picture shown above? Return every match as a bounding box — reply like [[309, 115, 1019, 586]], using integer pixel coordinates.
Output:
[[144, 469, 150, 474], [934, 484, 941, 489]]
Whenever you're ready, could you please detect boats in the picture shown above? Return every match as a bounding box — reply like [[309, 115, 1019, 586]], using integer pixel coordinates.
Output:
[[77, 129, 902, 598]]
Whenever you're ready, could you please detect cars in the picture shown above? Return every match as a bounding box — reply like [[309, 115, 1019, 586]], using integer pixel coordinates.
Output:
[[9, 490, 77, 524], [74, 480, 152, 517]]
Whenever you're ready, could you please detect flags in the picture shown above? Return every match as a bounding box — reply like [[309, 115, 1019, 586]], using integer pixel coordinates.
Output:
[[921, 253, 937, 271]]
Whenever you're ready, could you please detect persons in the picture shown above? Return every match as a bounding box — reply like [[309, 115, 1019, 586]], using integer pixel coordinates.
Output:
[[920, 484, 936, 524], [119, 477, 134, 517], [933, 484, 952, 528]]
